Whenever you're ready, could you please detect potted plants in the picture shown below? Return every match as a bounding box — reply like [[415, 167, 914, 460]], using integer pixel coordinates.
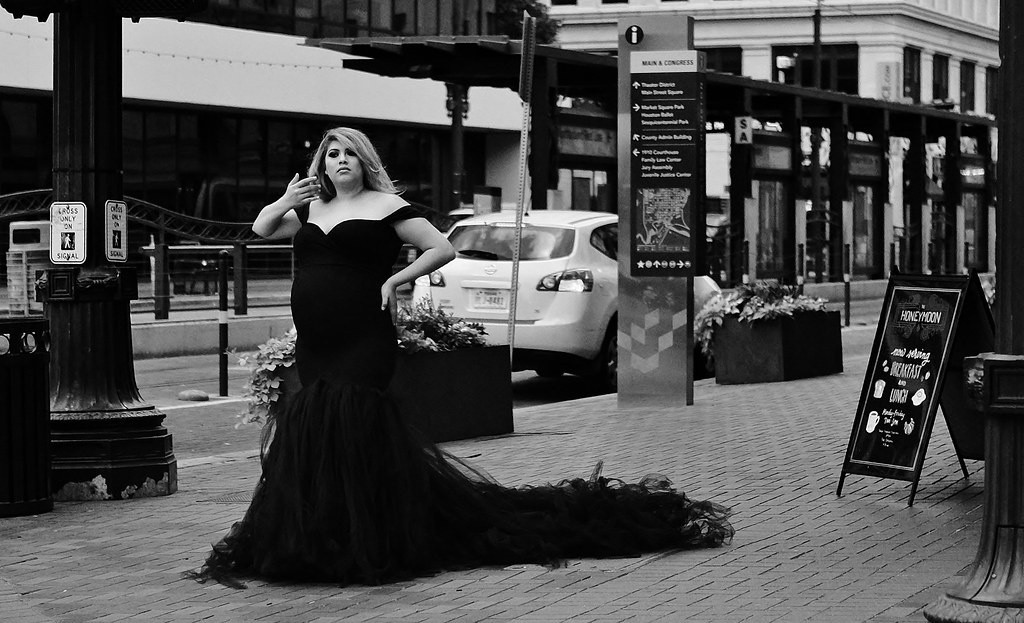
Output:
[[224, 291, 515, 444], [693, 275, 844, 386]]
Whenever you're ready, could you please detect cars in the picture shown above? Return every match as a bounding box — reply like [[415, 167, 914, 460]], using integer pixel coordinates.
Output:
[[409, 210, 619, 391]]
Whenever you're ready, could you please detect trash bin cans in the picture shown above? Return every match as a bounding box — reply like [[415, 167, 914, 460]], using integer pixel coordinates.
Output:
[[6, 219, 52, 313], [0, 314, 53, 516]]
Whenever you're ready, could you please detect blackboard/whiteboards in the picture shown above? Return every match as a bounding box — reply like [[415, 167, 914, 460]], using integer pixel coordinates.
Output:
[[845, 269, 996, 484]]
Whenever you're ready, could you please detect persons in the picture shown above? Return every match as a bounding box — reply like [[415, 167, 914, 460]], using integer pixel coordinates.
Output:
[[251, 128, 456, 587]]
